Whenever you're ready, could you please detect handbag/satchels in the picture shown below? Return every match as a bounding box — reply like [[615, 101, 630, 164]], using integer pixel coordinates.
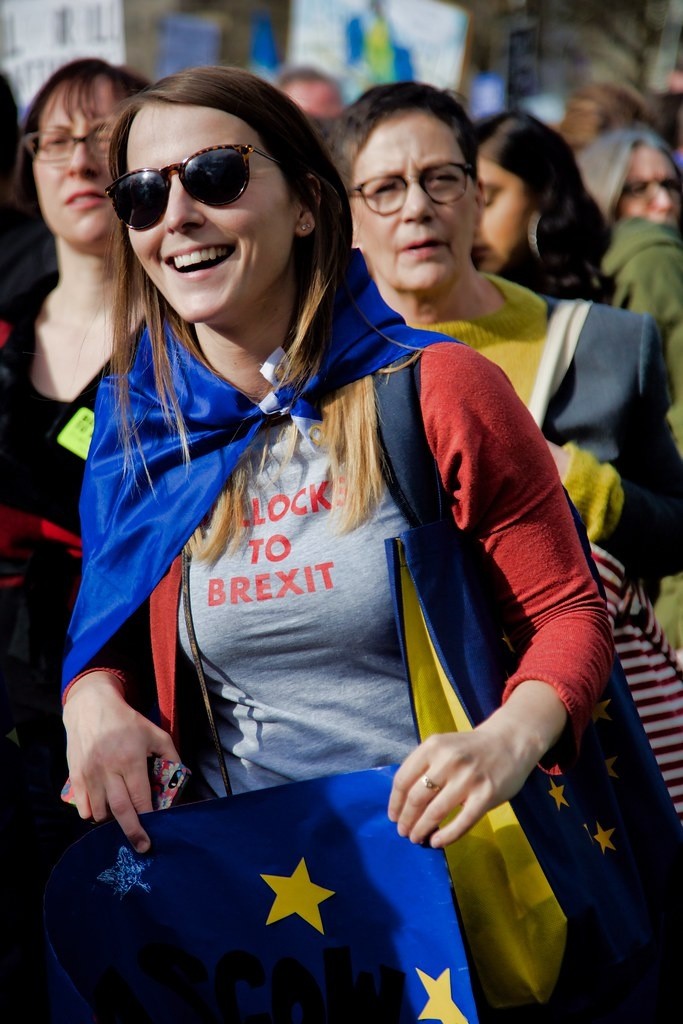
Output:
[[383, 338, 683, 1023]]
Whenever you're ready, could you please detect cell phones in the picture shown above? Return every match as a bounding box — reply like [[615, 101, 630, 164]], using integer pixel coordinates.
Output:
[[61, 755, 190, 812]]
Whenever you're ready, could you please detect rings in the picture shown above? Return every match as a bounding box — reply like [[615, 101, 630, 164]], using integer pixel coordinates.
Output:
[[421, 775, 440, 792]]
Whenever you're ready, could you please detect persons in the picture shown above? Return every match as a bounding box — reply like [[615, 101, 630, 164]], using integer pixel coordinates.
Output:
[[0, 59, 683, 1024]]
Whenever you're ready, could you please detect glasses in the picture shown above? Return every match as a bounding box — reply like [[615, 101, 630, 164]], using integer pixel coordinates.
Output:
[[621, 176, 683, 198], [348, 163, 472, 215], [22, 127, 115, 161], [105, 144, 282, 230]]
